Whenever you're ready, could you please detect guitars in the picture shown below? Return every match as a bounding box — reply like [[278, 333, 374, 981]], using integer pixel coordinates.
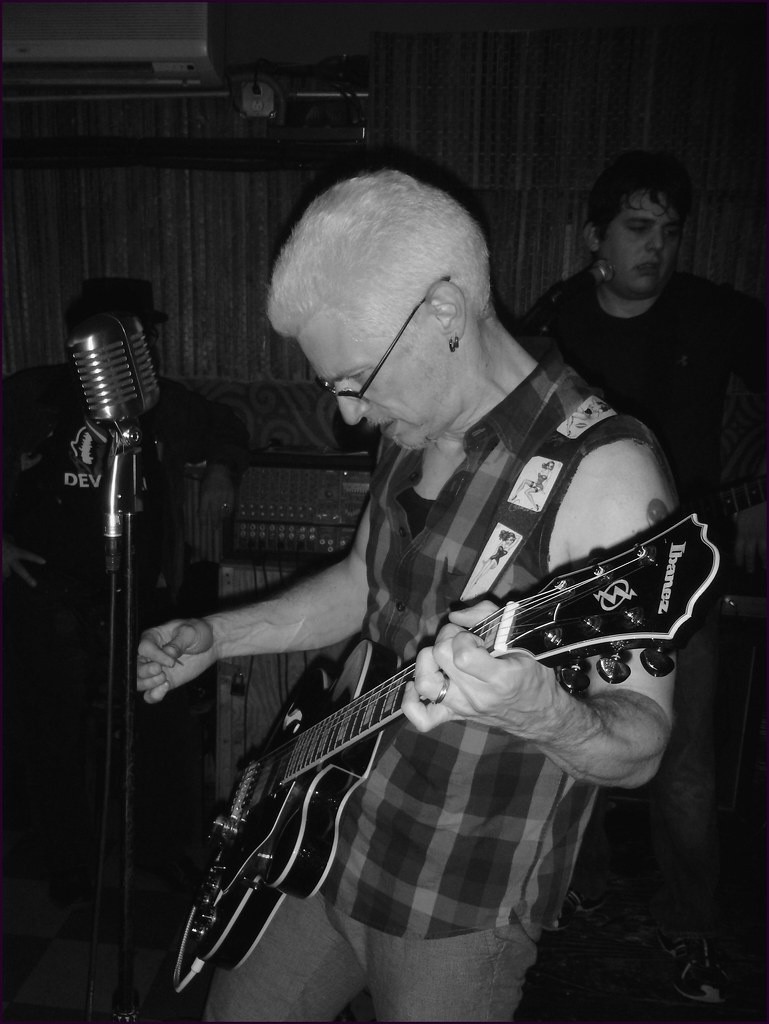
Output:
[[176, 509, 722, 995]]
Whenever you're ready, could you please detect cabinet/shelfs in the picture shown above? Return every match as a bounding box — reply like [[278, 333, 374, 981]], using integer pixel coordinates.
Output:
[[216, 561, 352, 802]]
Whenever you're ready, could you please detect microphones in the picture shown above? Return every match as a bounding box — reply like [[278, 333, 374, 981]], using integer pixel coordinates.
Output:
[[68, 309, 159, 569], [547, 260, 614, 307]]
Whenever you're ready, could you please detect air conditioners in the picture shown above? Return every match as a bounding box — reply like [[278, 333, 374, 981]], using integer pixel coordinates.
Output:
[[0, 0, 227, 86]]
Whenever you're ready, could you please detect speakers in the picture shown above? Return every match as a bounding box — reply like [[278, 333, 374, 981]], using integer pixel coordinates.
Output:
[[608, 642, 759, 813], [213, 560, 355, 813]]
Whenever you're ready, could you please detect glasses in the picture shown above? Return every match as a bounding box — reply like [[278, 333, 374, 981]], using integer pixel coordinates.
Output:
[[314, 275, 451, 405]]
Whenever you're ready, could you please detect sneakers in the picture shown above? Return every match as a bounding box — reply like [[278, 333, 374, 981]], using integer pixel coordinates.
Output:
[[537, 871, 614, 933], [654, 931, 731, 1009]]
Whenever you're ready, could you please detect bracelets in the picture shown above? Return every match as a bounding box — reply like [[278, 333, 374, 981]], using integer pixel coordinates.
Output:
[[434, 671, 450, 704]]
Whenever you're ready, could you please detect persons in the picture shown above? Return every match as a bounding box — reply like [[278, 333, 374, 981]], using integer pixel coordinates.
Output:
[[530, 155, 767, 1002], [134, 171, 678, 1022], [1, 279, 250, 887]]
